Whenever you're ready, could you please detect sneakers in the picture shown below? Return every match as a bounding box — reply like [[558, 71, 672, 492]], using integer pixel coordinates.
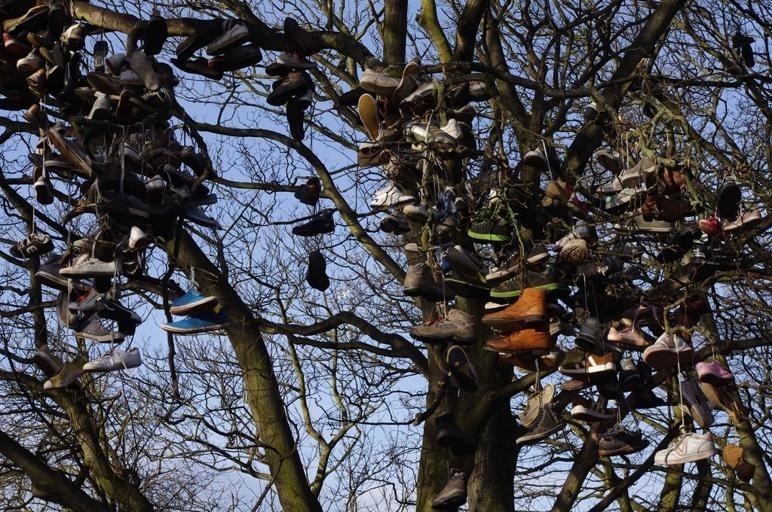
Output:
[[0, 0, 233, 342], [82, 347, 142, 371], [654, 432, 714, 465], [402, 78, 762, 441], [43, 356, 88, 390], [232, 14, 316, 143]]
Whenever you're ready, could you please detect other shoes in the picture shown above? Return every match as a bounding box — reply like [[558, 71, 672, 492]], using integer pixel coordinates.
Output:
[[723, 445, 756, 483], [293, 177, 320, 206], [306, 252, 331, 292], [430, 472, 467, 510], [335, 72, 401, 235], [293, 213, 335, 236]]
